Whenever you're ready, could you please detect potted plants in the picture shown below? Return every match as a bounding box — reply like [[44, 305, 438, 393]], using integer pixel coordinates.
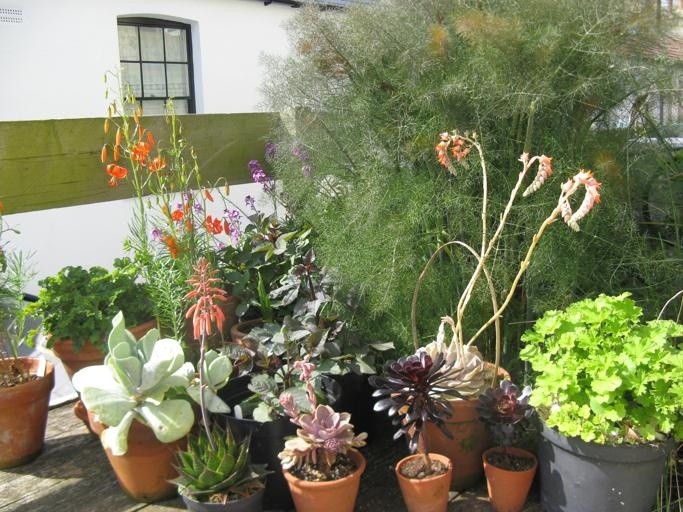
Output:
[[399, 342, 512, 488], [520, 290, 683, 509], [367, 350, 467, 511], [472, 377, 543, 510], [41, 65, 374, 510], [0, 218, 54, 470]]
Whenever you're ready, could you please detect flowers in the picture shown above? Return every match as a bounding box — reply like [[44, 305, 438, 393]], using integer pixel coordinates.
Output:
[[432, 127, 602, 235]]
